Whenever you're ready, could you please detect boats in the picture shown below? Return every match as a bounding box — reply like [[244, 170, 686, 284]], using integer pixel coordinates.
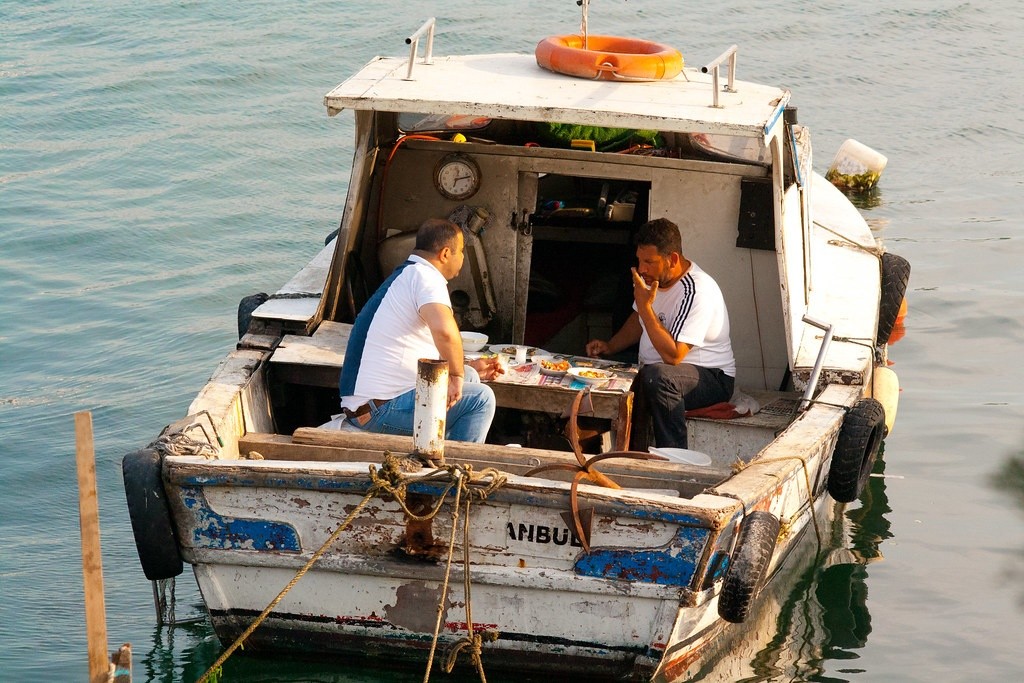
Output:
[[119, 17, 909, 676]]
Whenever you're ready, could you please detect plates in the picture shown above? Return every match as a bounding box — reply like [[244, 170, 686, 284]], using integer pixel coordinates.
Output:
[[567, 367, 617, 385], [489, 345, 550, 359], [531, 355, 572, 376]]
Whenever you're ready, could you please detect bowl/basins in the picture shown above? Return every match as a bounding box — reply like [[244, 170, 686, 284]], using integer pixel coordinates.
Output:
[[459, 331, 489, 352]]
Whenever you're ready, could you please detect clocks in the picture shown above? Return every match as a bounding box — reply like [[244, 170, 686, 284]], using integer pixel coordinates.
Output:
[[433, 152, 483, 201]]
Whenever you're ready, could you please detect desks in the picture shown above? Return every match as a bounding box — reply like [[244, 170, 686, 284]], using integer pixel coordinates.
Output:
[[479, 345, 642, 452]]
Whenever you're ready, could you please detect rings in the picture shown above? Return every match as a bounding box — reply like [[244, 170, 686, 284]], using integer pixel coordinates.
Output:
[[633, 284, 637, 287]]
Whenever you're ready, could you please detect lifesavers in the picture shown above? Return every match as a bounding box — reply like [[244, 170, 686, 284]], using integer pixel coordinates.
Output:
[[718, 509, 779, 622], [824, 396, 885, 502], [875, 249, 911, 344], [238, 291, 271, 343], [535, 33, 685, 81], [121, 449, 184, 582]]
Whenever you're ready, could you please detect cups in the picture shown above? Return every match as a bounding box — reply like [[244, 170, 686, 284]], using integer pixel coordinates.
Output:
[[614, 203, 636, 222], [516, 346, 528, 364]]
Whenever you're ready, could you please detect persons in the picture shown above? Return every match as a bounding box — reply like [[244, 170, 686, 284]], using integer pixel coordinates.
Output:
[[585, 217, 736, 452], [338, 219, 505, 446]]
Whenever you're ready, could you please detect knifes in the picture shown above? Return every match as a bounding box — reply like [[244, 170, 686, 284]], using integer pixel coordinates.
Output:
[[603, 363, 632, 371]]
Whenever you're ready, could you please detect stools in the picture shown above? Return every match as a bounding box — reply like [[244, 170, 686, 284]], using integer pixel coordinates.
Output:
[[264, 321, 354, 436], [682, 389, 803, 467]]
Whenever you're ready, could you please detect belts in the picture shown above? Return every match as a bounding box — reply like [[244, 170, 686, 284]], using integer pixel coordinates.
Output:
[[345, 400, 389, 419]]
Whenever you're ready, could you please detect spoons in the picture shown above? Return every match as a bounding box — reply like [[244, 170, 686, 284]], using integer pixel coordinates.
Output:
[[553, 355, 574, 359]]
